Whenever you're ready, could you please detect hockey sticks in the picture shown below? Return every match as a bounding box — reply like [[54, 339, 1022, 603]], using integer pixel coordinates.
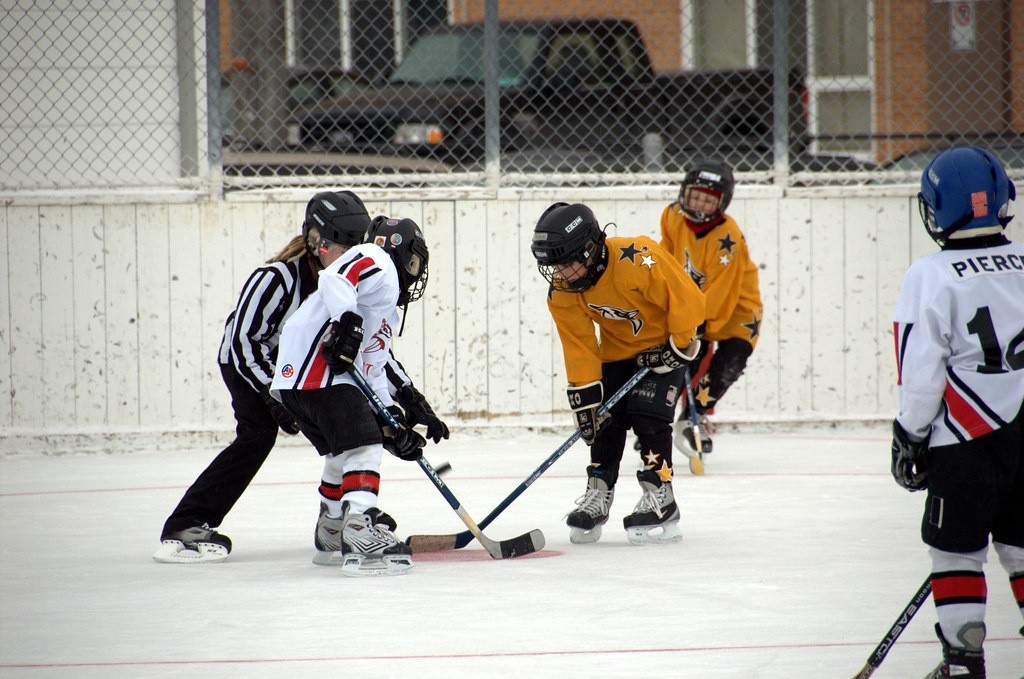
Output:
[[405, 365, 652, 553], [321, 332, 546, 561], [684, 366, 705, 476], [854, 573, 934, 679]]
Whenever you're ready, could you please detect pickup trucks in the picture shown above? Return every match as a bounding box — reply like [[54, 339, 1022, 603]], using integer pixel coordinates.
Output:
[[278, 16, 814, 182]]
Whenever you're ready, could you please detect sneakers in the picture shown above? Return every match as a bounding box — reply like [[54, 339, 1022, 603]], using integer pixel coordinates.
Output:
[[341, 500, 414, 576], [154, 523, 233, 563], [311, 502, 345, 564], [566, 465, 615, 544], [673, 405, 713, 460], [924, 621, 986, 679], [623, 470, 682, 545]]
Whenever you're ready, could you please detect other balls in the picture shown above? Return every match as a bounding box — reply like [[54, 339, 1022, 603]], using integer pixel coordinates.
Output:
[[435, 463, 451, 475]]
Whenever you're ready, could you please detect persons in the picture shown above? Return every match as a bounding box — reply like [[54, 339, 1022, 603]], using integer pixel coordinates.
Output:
[[655, 160, 764, 460], [531, 202, 706, 546], [891, 146, 1024, 679], [153, 191, 450, 564], [268, 215, 430, 576]]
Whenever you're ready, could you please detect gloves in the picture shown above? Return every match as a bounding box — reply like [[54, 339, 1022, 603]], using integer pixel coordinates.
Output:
[[262, 389, 299, 434], [890, 418, 932, 492], [567, 380, 603, 446], [395, 385, 450, 444], [636, 335, 701, 374], [374, 405, 427, 461], [321, 311, 365, 375]]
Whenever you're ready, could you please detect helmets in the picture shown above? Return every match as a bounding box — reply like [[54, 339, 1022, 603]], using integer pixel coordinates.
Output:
[[919, 145, 1016, 239], [302, 189, 371, 253], [675, 160, 734, 224], [363, 214, 428, 307], [532, 203, 609, 295]]
